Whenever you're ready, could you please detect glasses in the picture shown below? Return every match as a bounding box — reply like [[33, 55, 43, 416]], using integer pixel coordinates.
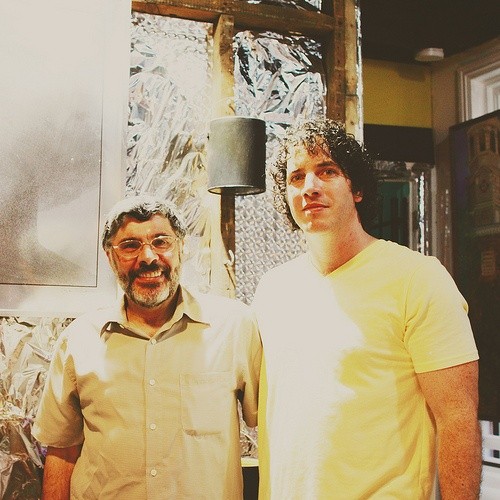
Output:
[[109, 235, 178, 260]]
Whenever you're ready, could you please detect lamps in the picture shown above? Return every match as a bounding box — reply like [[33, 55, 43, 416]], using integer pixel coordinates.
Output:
[[413, 47, 444, 62]]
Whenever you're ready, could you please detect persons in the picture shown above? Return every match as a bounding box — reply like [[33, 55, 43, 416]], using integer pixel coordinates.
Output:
[[240, 112, 485, 500], [32, 195, 269, 500]]
